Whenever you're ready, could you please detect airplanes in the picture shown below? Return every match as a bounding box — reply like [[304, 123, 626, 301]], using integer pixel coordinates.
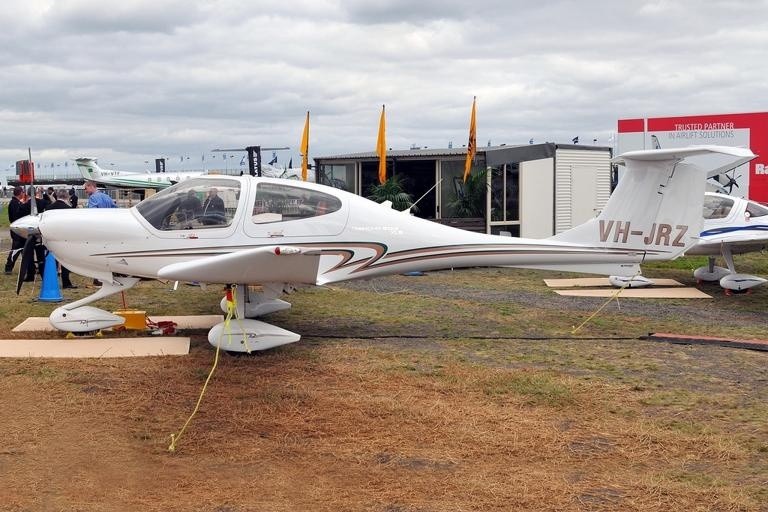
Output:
[[74, 155, 208, 195], [683, 170, 768, 292], [10, 144, 758, 354]]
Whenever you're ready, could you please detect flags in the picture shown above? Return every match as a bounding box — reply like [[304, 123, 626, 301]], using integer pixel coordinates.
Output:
[[300, 111, 309, 181], [462, 98, 477, 184], [375, 106, 387, 184]]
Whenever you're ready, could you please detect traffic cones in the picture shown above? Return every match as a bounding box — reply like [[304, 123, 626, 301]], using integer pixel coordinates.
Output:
[[36, 251, 64, 303]]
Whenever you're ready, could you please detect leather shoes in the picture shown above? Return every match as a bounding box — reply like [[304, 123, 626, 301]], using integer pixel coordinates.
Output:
[[4, 265, 102, 288]]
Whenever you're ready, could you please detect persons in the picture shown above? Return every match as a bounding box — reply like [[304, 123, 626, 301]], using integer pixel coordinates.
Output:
[[4, 179, 119, 290], [200, 187, 226, 224], [175, 189, 203, 229]]
